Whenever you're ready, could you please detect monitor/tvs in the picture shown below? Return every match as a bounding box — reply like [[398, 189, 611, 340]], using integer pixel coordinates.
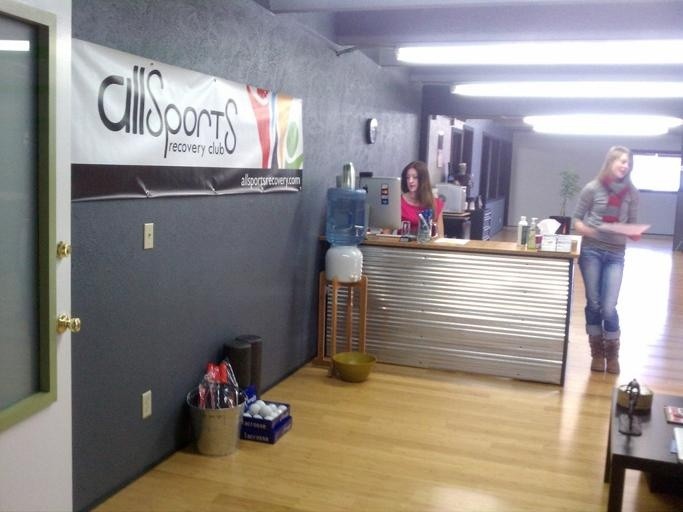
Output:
[[336, 175, 401, 234]]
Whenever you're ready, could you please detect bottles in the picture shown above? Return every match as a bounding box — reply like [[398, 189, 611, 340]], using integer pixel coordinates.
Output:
[[470, 199, 475, 210], [517, 215, 529, 247]]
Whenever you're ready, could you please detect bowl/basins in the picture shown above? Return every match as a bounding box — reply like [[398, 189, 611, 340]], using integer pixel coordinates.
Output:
[[332, 351, 377, 383]]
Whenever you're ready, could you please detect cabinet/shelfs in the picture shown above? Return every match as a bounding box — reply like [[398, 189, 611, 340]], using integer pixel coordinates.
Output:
[[469, 208, 492, 241], [484, 196, 506, 235]]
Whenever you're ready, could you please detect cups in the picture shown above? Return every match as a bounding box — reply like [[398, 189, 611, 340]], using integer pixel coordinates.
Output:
[[417, 218, 439, 244], [464, 201, 468, 210], [341, 161, 355, 190], [459, 162, 467, 175], [571, 240, 577, 253], [535, 235, 542, 248]]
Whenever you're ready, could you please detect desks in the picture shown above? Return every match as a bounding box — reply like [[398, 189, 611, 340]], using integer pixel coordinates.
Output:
[[601, 390, 681, 510]]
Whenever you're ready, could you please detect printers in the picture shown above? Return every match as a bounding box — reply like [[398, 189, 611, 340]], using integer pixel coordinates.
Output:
[[436, 183, 467, 213]]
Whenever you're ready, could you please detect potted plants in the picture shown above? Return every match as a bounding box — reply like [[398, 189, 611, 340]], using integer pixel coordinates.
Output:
[[547, 170, 581, 236]]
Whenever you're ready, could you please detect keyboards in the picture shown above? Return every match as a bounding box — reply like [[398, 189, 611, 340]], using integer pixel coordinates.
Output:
[[376, 234, 417, 238]]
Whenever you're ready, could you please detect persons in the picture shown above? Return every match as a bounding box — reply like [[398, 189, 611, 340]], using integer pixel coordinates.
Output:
[[379, 160, 444, 241], [573, 146, 641, 374]]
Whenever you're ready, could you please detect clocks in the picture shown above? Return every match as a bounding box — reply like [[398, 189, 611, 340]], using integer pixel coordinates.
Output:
[[367, 117, 377, 144]]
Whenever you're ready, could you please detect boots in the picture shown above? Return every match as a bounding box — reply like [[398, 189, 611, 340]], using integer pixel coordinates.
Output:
[[589, 342, 606, 372], [605, 339, 620, 373]]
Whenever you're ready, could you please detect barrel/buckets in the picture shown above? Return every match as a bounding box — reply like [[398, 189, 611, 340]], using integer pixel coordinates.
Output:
[[326, 188, 369, 245], [187, 394, 245, 456]]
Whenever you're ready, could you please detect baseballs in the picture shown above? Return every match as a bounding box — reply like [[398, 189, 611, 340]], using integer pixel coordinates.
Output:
[[243, 399, 291, 441]]
